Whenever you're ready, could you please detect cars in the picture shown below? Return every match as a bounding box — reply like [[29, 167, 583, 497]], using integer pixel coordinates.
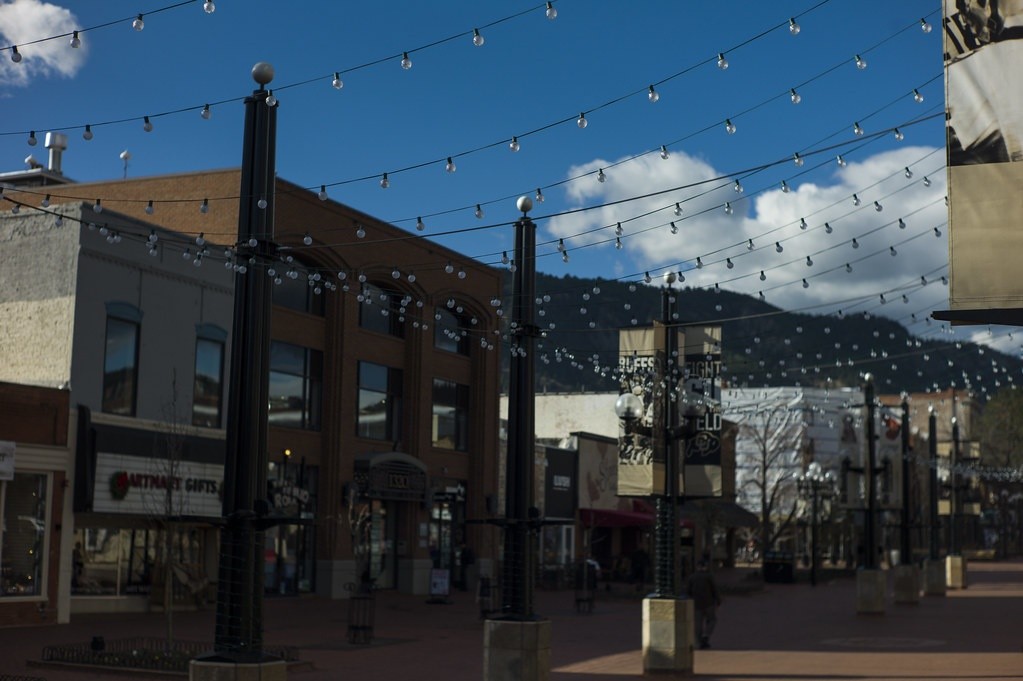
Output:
[[764, 551, 793, 582], [962, 542, 999, 561]]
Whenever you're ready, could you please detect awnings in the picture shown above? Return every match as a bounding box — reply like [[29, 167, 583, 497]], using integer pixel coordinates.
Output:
[[580, 508, 695, 530]]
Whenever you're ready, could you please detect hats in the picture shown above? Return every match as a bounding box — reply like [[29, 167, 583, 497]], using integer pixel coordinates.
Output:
[[696, 560, 708, 567]]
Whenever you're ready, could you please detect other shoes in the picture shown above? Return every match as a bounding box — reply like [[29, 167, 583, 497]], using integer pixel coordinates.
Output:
[[697, 636, 712, 650]]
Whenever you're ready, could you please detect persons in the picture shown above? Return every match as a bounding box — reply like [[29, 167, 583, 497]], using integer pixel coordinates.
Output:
[[687, 560, 720, 650], [573, 554, 601, 616], [73, 542, 84, 577], [629, 548, 650, 587]]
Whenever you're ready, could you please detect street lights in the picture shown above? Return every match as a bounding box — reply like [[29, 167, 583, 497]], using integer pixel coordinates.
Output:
[[951, 417, 962, 556], [928, 406, 942, 561], [792, 462, 838, 587], [485, 197, 547, 621], [899, 391, 913, 564], [615, 271, 707, 600], [861, 372, 881, 570], [1001, 490, 1009, 558], [192, 63, 280, 662]]
